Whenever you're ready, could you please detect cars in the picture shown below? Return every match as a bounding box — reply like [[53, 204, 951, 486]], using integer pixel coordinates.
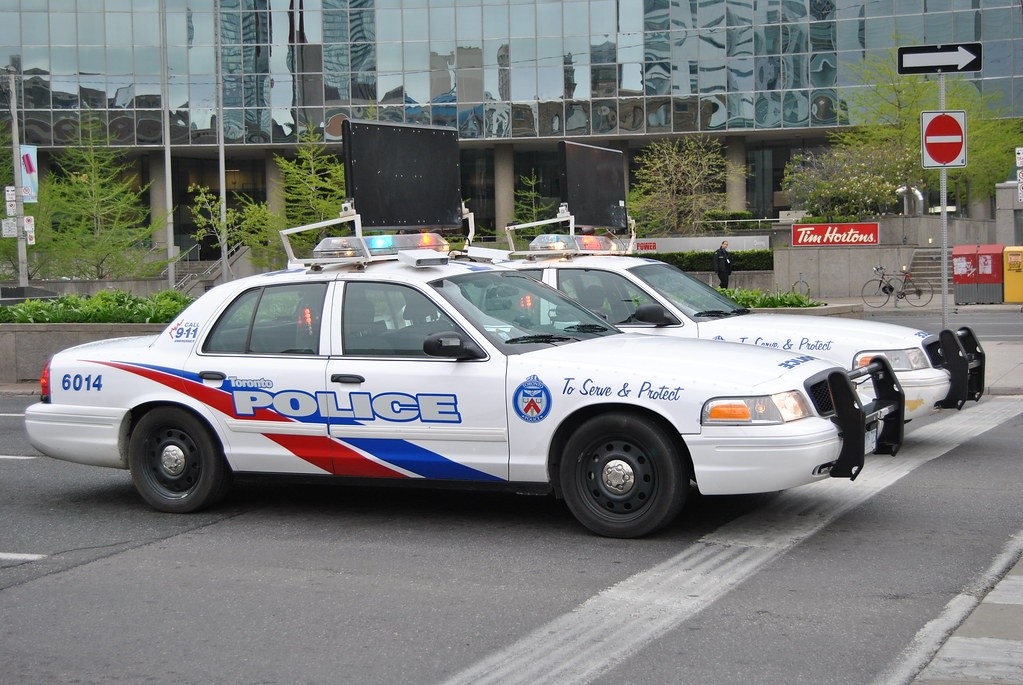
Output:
[[23, 227, 987, 543]]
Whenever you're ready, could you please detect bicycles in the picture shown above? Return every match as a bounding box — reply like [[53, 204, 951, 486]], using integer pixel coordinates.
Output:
[[860, 264, 934, 308]]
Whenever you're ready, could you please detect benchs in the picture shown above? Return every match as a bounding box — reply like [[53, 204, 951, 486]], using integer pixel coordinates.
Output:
[[216, 319, 297, 353]]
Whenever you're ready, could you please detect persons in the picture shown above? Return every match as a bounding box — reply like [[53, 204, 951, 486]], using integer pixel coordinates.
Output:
[[714, 241, 734, 289]]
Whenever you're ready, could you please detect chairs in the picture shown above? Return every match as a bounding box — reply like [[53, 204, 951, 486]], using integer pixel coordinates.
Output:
[[346, 298, 395, 351], [580, 285, 603, 311], [398, 294, 453, 346]]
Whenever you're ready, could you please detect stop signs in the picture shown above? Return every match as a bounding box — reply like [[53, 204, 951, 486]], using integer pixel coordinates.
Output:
[[921, 110, 968, 168]]
